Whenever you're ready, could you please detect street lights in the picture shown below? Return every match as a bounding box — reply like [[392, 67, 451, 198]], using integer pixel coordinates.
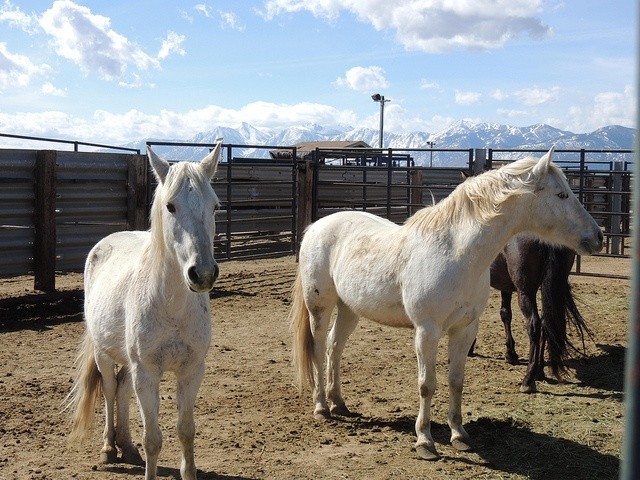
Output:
[[371, 93, 391, 148], [428, 141, 435, 168]]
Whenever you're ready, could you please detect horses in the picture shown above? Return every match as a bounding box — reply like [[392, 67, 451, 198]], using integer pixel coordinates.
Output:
[[459, 169, 597, 394], [59, 137, 223, 480], [285, 143, 605, 462]]
